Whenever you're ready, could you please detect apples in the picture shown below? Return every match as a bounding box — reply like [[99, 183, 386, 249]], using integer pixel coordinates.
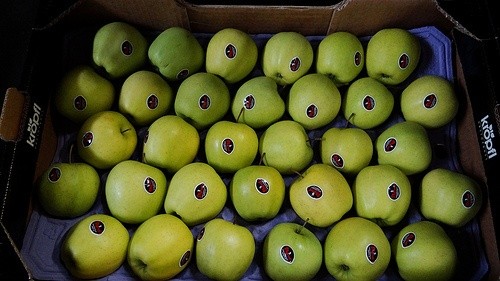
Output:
[[37, 22, 481, 281]]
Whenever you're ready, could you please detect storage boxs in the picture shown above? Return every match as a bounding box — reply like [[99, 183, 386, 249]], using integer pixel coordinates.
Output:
[[1, 0, 500, 281]]
